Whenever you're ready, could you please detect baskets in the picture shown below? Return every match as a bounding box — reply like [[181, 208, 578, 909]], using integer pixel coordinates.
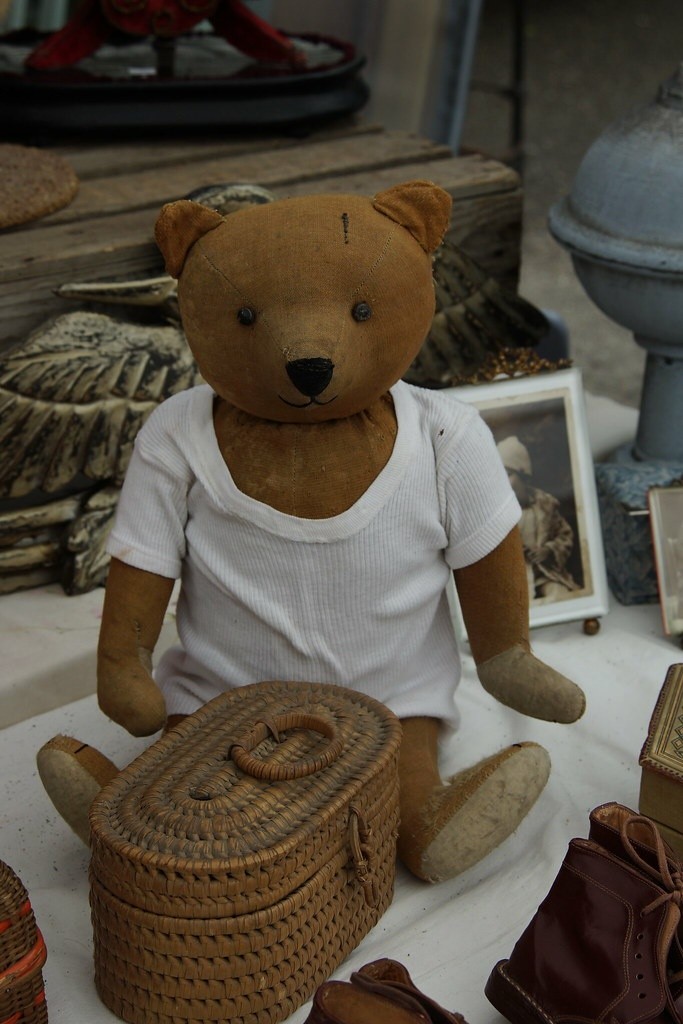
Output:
[[0, 860, 48, 1024]]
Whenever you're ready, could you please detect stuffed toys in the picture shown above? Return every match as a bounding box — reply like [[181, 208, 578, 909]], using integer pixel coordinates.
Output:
[[38, 182, 587, 884]]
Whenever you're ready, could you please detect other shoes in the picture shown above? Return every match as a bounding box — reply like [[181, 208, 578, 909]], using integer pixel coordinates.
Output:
[[303, 980, 431, 1024], [350, 957, 470, 1024]]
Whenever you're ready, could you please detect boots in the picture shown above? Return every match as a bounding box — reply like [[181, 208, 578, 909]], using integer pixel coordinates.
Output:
[[484, 838, 683, 1024], [589, 801, 683, 893]]
